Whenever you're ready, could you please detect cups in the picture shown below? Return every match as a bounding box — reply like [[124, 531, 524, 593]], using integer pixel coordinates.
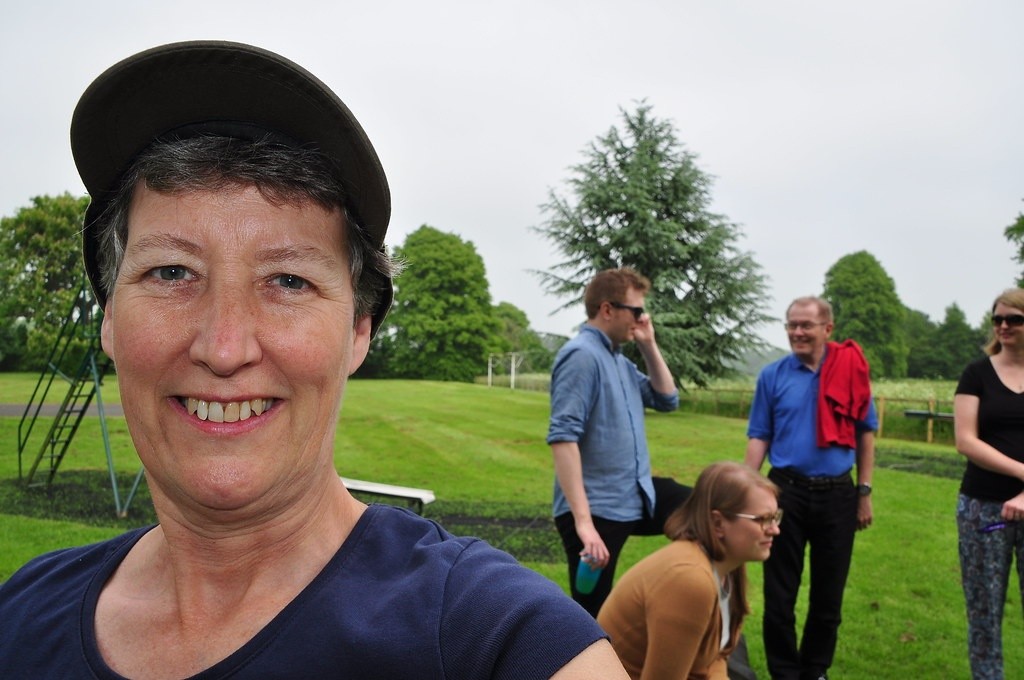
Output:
[[576, 554, 603, 593]]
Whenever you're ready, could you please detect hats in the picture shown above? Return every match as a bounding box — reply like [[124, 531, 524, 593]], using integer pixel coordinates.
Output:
[[69, 40, 393, 342]]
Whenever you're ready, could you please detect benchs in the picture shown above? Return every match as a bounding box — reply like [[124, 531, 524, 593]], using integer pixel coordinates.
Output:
[[343, 477, 435, 517], [905, 409, 954, 444]]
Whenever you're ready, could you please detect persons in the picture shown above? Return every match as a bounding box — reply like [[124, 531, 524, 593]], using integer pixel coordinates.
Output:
[[0, 40, 627, 680], [952, 289, 1024, 680], [745, 296, 879, 680], [547, 269, 780, 680]]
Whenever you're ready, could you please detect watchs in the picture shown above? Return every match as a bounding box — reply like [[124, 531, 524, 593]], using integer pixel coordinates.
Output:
[[856, 482, 871, 497]]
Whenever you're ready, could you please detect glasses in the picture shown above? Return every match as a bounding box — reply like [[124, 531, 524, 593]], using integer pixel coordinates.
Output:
[[598, 300, 645, 320], [784, 322, 826, 330], [992, 314, 1024, 327], [721, 511, 783, 530]]
